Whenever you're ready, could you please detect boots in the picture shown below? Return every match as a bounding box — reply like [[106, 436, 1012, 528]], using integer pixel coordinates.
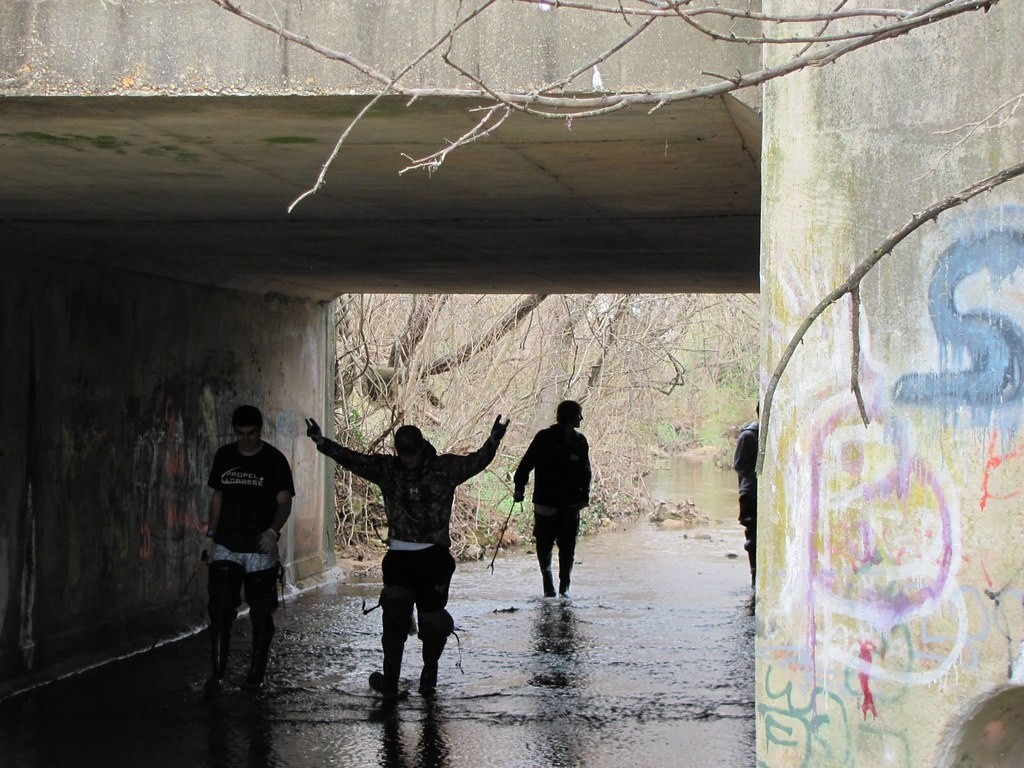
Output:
[[243, 631, 274, 688], [419, 636, 447, 696], [203, 630, 229, 694], [369, 634, 406, 699]]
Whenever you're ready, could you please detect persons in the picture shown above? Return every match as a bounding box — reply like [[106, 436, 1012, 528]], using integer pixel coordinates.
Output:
[[734, 402, 759, 617], [513, 401, 592, 599], [196, 406, 295, 690], [305, 414, 510, 698]]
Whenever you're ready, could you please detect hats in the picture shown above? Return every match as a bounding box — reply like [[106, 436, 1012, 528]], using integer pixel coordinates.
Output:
[[395, 424, 423, 456]]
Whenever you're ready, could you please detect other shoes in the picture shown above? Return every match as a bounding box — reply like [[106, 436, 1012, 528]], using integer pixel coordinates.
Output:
[[542, 573, 556, 598], [559, 573, 570, 598]]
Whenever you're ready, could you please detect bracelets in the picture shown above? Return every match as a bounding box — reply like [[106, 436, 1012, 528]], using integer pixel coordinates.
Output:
[[207, 530, 216, 536]]
